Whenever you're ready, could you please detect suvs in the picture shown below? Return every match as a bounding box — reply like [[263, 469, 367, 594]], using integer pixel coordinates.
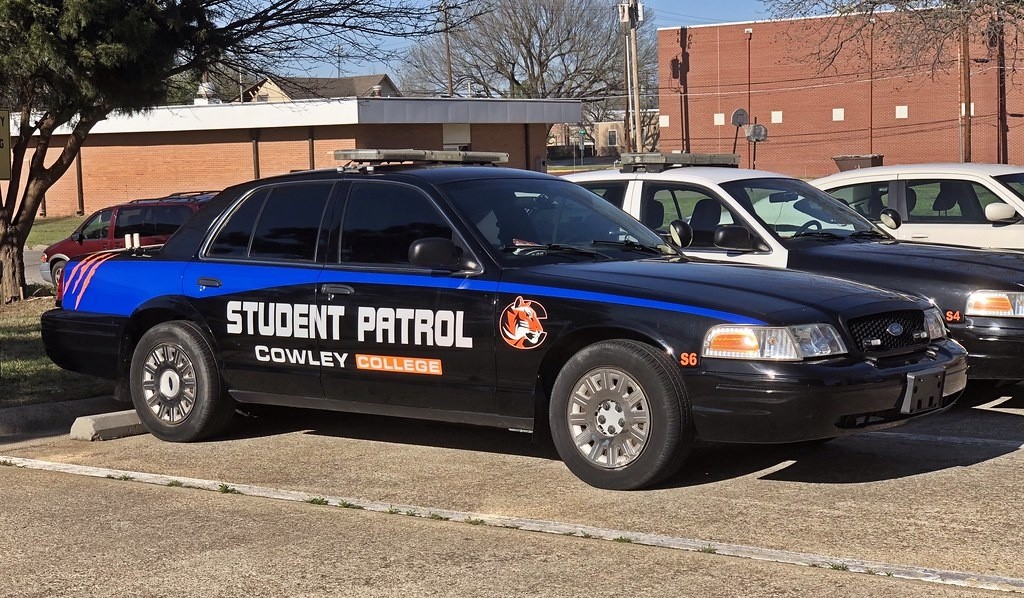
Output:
[[40, 189, 224, 291]]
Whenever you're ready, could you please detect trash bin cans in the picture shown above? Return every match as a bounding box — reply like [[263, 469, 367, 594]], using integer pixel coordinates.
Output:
[[832, 154, 883, 172]]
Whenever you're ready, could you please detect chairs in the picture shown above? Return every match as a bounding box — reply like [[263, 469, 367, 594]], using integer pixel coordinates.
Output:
[[906, 188, 917, 216], [690, 197, 721, 230], [933, 189, 957, 216], [640, 199, 668, 240]]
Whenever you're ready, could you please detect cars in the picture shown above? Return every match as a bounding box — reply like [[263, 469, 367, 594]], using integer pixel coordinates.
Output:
[[40, 150, 972, 492], [687, 162, 1024, 253], [463, 151, 1023, 408]]
